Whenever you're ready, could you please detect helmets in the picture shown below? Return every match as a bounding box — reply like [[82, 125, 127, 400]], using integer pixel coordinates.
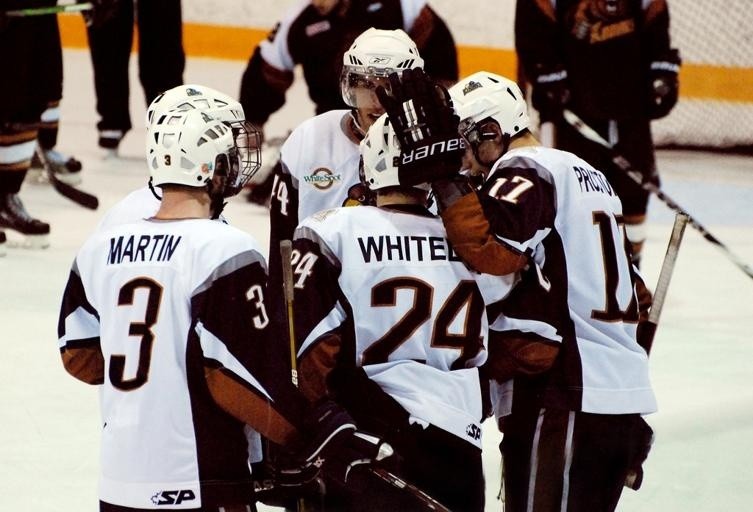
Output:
[[141, 83, 263, 196], [338, 26, 429, 135], [444, 71, 532, 169], [357, 112, 436, 196]]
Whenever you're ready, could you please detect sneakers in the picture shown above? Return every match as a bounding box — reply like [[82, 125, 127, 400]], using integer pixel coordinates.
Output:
[[98, 128, 126, 149], [0, 193, 50, 235], [31, 149, 82, 173], [630, 257, 653, 312]]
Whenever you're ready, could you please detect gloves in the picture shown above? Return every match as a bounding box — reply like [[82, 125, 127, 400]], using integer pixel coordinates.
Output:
[[247, 399, 397, 509], [529, 47, 572, 115], [646, 47, 682, 119], [373, 66, 468, 188]]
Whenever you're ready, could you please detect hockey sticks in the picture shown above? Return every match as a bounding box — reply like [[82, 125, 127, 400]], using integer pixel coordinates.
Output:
[[35, 140, 97, 209]]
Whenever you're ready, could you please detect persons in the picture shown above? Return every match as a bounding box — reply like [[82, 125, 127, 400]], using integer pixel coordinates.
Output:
[[239, 0, 459, 150], [0, 0, 81, 247], [515, 0, 681, 270], [95, 27, 657, 512], [76, 1, 185, 159], [57, 85, 400, 512]]
